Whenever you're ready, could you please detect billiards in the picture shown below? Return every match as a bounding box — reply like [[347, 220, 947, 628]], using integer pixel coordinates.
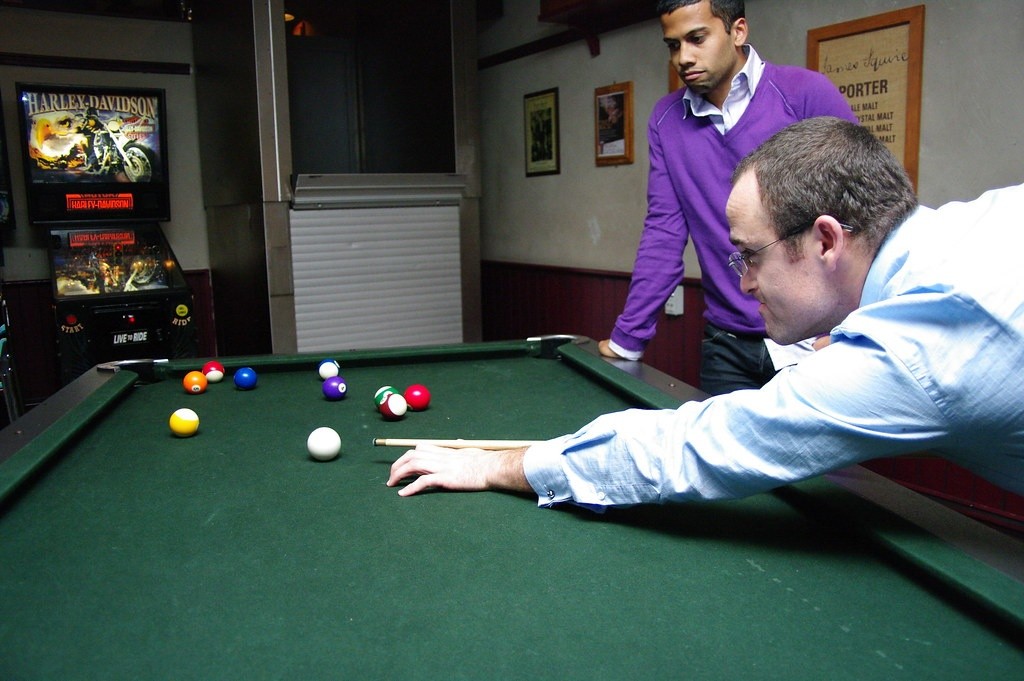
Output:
[[163, 355, 432, 464]]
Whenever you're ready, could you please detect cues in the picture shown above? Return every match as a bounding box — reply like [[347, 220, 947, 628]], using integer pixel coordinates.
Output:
[[372, 436, 548, 450]]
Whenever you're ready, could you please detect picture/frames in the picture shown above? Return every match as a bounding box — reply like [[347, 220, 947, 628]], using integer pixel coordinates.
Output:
[[525, 87, 561, 178], [594, 80, 634, 166]]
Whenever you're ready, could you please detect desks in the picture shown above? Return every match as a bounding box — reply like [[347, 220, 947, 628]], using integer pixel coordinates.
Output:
[[0, 334, 1024, 681]]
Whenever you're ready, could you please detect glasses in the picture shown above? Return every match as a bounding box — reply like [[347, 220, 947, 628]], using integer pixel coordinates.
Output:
[[729, 218, 854, 277]]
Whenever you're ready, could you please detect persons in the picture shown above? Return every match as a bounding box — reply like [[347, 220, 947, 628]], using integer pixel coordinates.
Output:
[[386, 115, 1024, 513], [597, 0, 861, 395]]
